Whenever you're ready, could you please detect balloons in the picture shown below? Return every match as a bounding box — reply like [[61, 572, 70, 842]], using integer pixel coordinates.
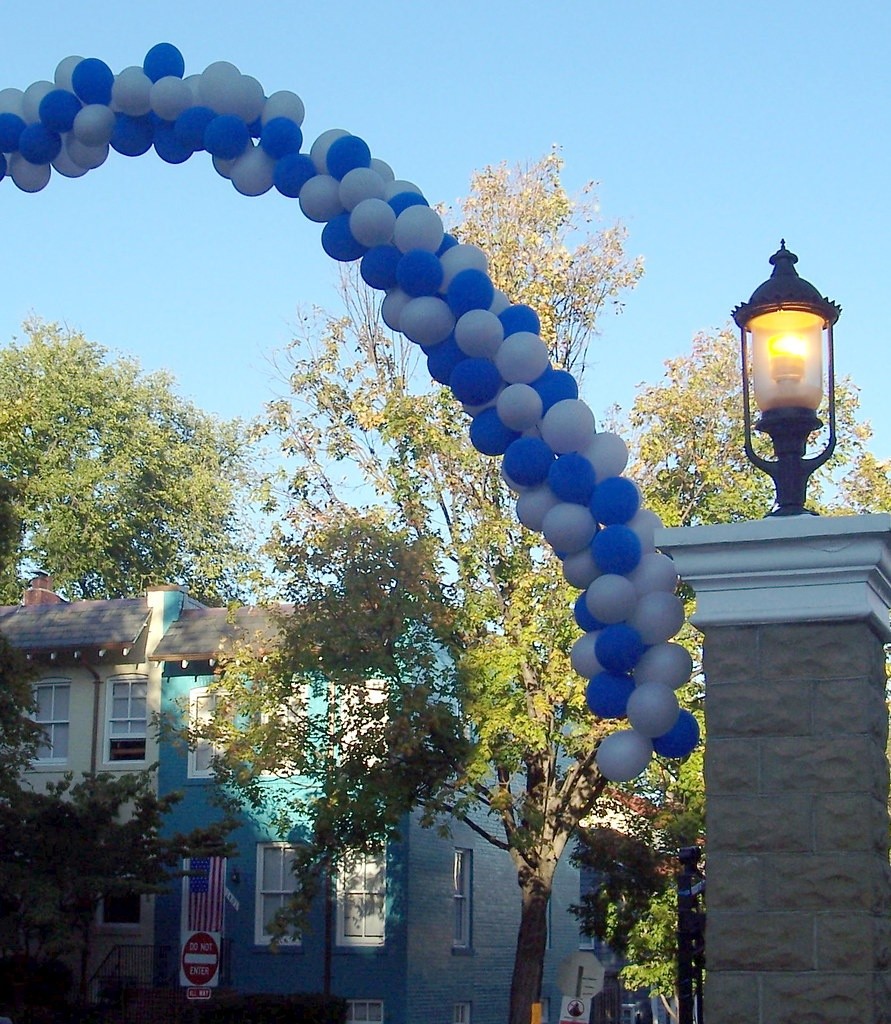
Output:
[[0, 44, 701, 780]]
